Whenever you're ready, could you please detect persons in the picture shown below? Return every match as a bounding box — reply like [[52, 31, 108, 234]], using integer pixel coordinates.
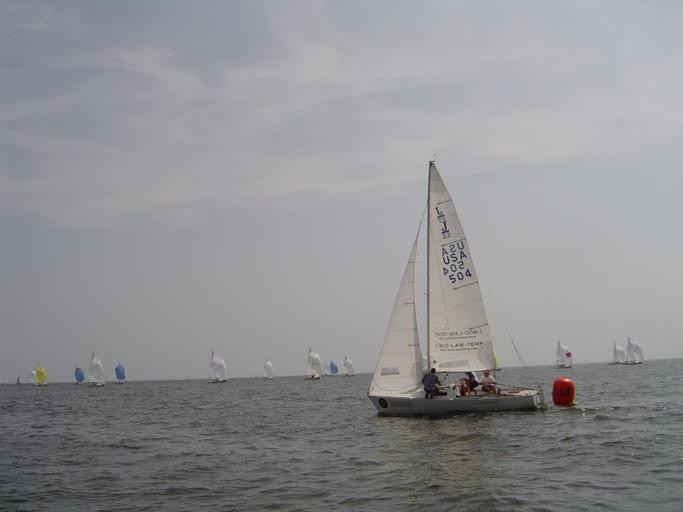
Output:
[[479, 370, 496, 393], [459, 371, 478, 389], [420, 368, 440, 399]]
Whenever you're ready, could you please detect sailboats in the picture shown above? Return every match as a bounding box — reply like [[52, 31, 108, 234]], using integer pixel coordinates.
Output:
[[207, 351, 227, 384], [16, 360, 47, 387], [303, 346, 354, 381], [262, 356, 274, 380], [365, 159, 545, 416], [552, 339, 572, 369], [74, 352, 125, 387], [608, 336, 643, 365]]
